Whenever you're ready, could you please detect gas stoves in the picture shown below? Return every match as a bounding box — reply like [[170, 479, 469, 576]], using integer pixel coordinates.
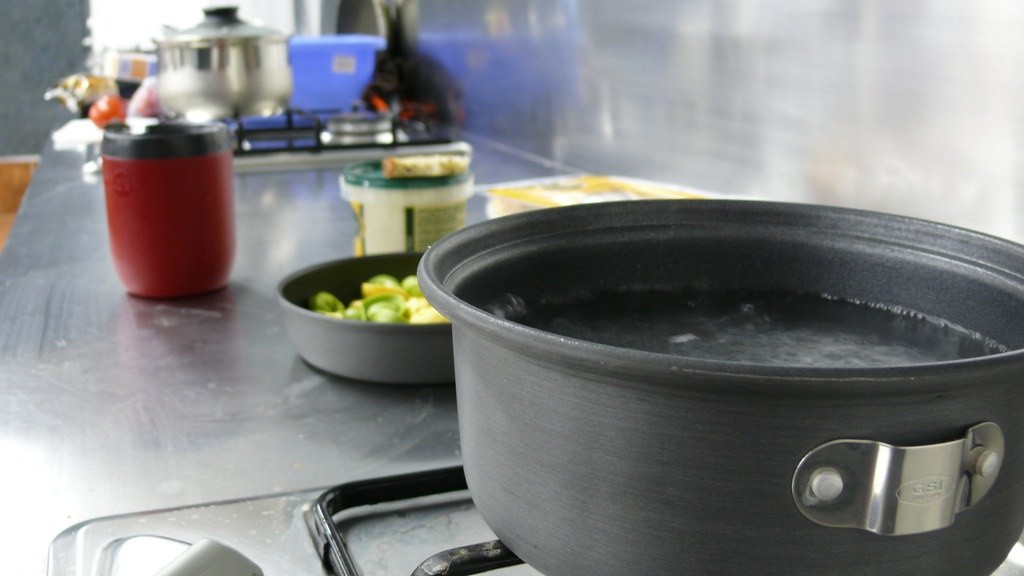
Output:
[[0, 126, 1024, 576]]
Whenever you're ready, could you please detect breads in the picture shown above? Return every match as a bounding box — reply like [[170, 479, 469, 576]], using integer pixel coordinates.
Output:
[[382, 152, 469, 177]]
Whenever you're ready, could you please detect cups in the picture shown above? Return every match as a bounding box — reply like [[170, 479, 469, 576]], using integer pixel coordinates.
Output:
[[337, 160, 475, 257], [102, 124, 236, 297]]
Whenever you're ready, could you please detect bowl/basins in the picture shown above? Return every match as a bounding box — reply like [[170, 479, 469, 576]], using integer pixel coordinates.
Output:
[[287, 34, 388, 113], [277, 252, 455, 382]]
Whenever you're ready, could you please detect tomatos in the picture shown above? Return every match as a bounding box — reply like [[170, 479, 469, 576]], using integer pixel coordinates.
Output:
[[88, 94, 126, 127]]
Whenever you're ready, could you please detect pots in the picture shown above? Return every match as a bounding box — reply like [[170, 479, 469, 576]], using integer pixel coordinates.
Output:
[[418, 200, 1024, 576], [152, 5, 292, 121]]
